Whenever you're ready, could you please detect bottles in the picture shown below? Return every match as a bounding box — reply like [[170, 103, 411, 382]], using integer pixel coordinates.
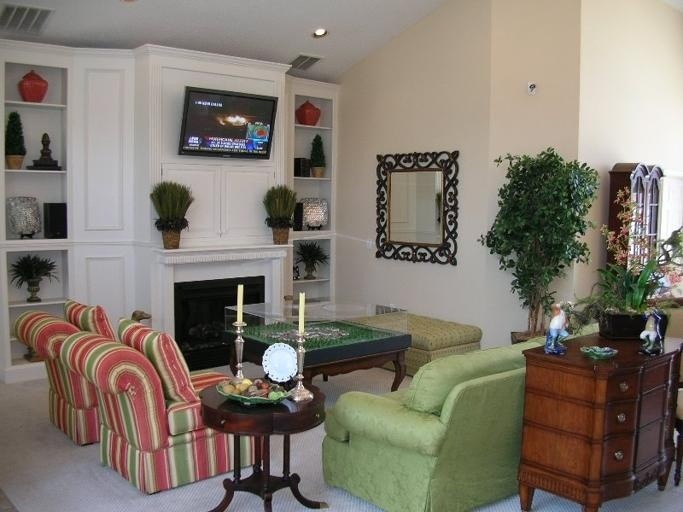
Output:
[[17, 71, 48, 103], [295, 100, 321, 126]]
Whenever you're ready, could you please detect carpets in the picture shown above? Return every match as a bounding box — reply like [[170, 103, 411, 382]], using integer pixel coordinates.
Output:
[[0, 359, 682, 511]]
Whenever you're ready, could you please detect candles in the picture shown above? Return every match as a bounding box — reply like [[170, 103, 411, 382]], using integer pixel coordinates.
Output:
[[298, 291, 305, 334], [236, 283, 243, 322]]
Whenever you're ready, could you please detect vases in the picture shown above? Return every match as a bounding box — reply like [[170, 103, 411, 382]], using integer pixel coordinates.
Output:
[[597, 308, 671, 342]]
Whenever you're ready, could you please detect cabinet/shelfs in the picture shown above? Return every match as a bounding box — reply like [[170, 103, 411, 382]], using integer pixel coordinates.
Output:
[[0, 37, 341, 386], [517, 329, 682, 511], [606, 162, 666, 288]]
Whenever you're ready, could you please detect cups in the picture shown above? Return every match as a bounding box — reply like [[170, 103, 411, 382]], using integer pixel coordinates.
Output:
[[294, 158, 311, 177], [293, 203, 302, 230]]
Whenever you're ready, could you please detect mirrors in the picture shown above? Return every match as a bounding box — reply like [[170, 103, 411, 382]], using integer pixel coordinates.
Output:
[[375, 150, 460, 265]]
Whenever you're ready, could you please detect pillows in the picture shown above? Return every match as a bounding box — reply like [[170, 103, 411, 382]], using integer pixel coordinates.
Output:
[[402, 320, 599, 412]]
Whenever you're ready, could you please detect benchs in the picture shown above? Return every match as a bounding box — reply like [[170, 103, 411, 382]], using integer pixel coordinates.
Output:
[[344, 310, 482, 378]]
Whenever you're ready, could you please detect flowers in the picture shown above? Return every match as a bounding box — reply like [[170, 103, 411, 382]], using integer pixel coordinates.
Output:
[[558, 184, 683, 337]]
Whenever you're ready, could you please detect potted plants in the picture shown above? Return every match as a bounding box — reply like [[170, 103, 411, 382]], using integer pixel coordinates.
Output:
[[148, 179, 194, 249], [295, 240, 330, 280], [310, 133, 327, 178], [261, 183, 297, 245], [8, 254, 61, 302], [4, 111, 27, 170], [477, 145, 600, 345]]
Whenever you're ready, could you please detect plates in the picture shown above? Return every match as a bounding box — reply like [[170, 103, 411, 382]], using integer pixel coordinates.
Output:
[[581, 345, 618, 362], [261, 342, 296, 384], [215, 380, 289, 406]]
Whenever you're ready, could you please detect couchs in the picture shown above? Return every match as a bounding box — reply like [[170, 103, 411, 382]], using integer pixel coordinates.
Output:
[[320, 363, 526, 512]]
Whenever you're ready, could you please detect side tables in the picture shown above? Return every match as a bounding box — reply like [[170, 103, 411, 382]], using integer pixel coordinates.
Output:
[[199, 381, 329, 510]]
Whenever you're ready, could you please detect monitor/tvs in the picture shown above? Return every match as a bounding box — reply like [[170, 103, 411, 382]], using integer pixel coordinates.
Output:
[[178, 85, 279, 160]]
